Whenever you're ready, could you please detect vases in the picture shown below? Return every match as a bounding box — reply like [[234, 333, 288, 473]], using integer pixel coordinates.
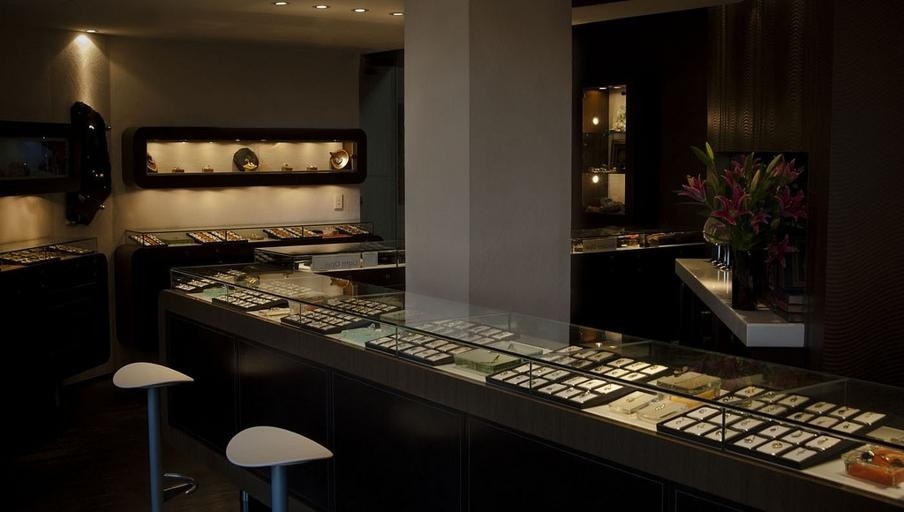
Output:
[[731, 248, 760, 312]]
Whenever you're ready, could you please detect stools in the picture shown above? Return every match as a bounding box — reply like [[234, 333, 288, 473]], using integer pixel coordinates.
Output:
[[112, 361, 335, 512]]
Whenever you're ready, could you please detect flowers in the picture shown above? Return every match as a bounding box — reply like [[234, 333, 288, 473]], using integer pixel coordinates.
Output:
[[672, 141, 809, 270]]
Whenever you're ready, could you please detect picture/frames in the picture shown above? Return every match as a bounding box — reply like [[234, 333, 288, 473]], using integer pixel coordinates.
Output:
[[609, 139, 624, 169]]
